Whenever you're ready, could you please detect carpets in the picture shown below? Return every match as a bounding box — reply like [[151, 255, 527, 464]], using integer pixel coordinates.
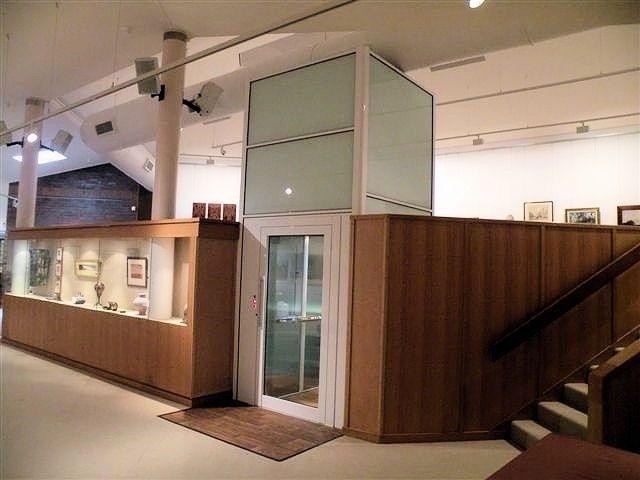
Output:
[[157, 399, 345, 461]]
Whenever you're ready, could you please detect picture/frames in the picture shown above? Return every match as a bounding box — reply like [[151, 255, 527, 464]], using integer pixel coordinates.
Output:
[[127, 256, 148, 288], [55, 263, 62, 277], [55, 247, 62, 261], [54, 280, 61, 294], [524, 201, 553, 223], [74, 260, 101, 279], [617, 205, 640, 226], [565, 208, 600, 226]]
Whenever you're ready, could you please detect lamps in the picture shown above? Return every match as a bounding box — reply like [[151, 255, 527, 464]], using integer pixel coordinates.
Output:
[[25, 123, 43, 144]]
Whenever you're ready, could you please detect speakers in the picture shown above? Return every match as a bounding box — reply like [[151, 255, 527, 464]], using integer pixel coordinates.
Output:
[[52, 129, 73, 155], [194, 81, 224, 119], [0, 120, 13, 147], [134, 57, 160, 96]]
